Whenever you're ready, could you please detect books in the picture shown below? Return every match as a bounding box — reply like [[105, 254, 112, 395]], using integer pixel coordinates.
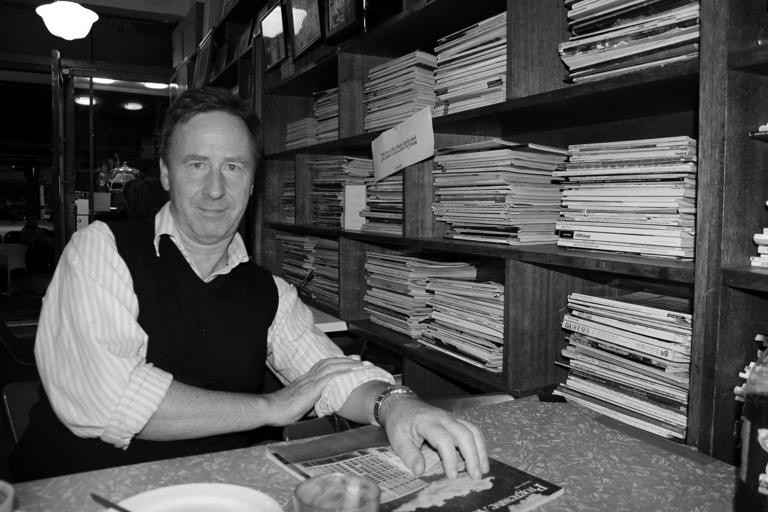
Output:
[[284, 12, 507, 151], [265, 422, 564, 512], [733, 125, 768, 402], [276, 233, 504, 374], [552, 278, 693, 439], [279, 155, 402, 235], [432, 136, 697, 261], [557, 0, 701, 84]]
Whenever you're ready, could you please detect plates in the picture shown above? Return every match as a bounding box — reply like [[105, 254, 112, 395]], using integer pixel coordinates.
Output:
[[104, 483, 283, 512]]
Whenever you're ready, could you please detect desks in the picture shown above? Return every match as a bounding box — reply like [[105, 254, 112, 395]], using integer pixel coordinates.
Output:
[[0, 389, 754, 510]]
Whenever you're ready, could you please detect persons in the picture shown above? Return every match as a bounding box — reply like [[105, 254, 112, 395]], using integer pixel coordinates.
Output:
[[7, 85, 491, 482]]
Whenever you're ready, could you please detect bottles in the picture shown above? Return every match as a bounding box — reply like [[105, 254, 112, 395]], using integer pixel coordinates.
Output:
[[734, 339, 767, 511]]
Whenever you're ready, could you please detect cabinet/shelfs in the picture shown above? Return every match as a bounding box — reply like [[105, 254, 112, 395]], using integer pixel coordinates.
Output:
[[170, 0, 768, 483]]
